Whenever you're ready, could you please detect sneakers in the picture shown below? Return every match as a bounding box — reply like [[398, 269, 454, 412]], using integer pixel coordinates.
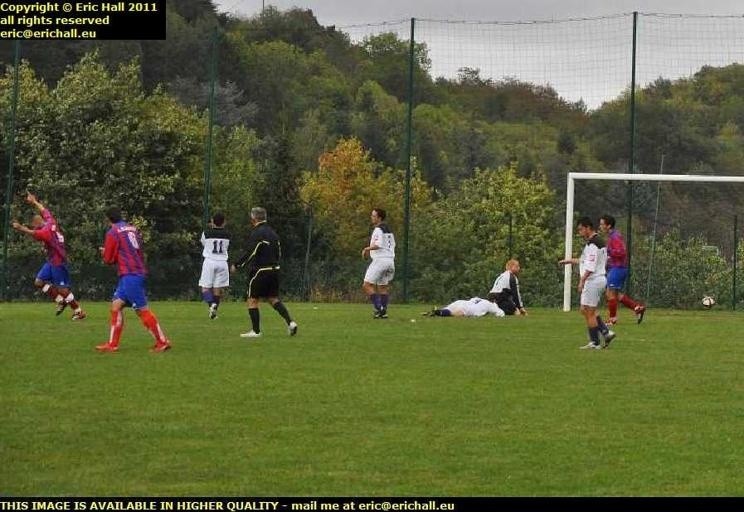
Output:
[[374, 306, 388, 318], [95, 343, 119, 353], [240, 329, 262, 338], [635, 305, 645, 324], [56, 298, 67, 315], [603, 319, 617, 326], [148, 339, 171, 352], [289, 321, 297, 336], [71, 312, 85, 320], [604, 330, 616, 347], [579, 341, 601, 350], [209, 303, 217, 319]]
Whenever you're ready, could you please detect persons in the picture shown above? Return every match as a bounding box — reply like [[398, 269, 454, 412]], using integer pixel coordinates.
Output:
[[601, 214, 646, 326], [558, 216, 615, 349], [490, 259, 527, 316], [424, 293, 505, 318], [231, 207, 298, 338], [95, 207, 172, 353], [198, 212, 231, 318], [12, 189, 86, 321], [361, 208, 396, 319]]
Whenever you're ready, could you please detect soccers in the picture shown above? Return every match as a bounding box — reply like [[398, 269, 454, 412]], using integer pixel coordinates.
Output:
[[702, 296, 716, 309]]
